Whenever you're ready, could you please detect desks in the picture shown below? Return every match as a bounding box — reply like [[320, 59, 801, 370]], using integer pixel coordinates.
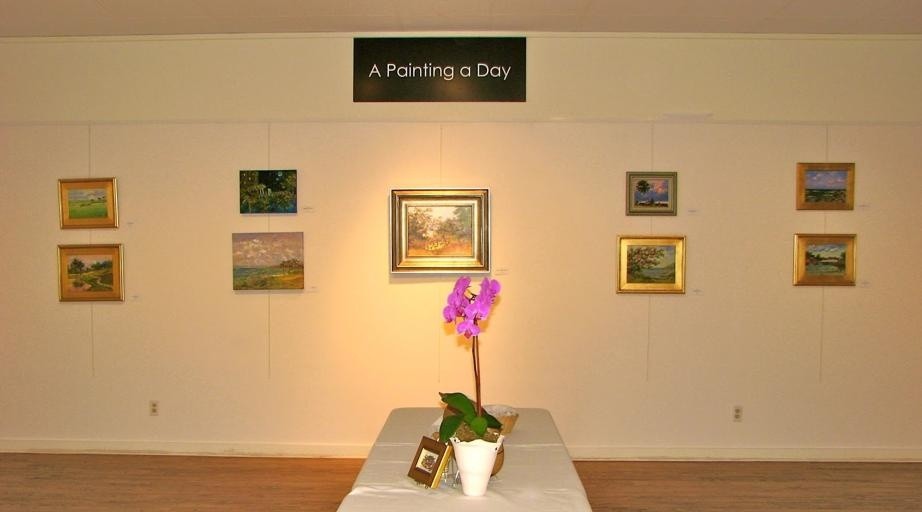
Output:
[[337, 408, 593, 511]]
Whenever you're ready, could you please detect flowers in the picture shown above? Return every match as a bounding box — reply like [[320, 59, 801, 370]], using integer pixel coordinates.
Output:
[[437, 277, 501, 442]]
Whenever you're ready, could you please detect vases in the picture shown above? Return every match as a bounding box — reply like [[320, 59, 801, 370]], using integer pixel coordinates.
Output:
[[448, 429, 504, 497]]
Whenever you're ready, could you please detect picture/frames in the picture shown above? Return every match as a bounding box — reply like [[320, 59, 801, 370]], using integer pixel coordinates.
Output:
[[58, 176, 118, 229], [625, 170, 677, 216], [797, 162, 855, 210], [793, 233, 855, 286], [617, 236, 688, 294], [56, 243, 125, 302], [407, 435, 451, 487], [387, 188, 492, 278]]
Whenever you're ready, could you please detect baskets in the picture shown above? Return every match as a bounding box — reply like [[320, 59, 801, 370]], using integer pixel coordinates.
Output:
[[487, 414, 518, 432]]
